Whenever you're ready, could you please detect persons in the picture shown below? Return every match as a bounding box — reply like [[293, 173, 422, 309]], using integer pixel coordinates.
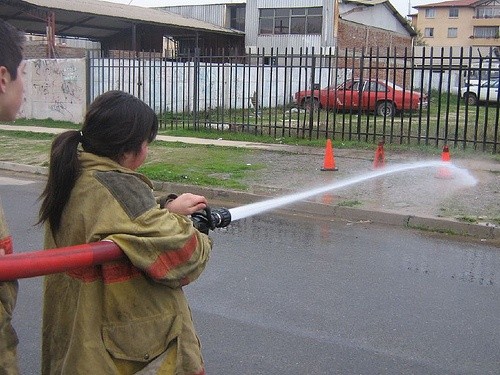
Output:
[[32, 89, 213, 375], [0, 17, 26, 375]]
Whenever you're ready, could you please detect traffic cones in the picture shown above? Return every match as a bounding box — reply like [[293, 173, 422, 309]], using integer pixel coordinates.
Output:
[[432, 146, 455, 180], [372, 141, 388, 174], [323, 139, 339, 170]]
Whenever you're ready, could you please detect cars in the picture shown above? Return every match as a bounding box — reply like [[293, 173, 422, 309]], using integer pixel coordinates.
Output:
[[293, 78, 420, 118], [461, 77, 499, 104]]
